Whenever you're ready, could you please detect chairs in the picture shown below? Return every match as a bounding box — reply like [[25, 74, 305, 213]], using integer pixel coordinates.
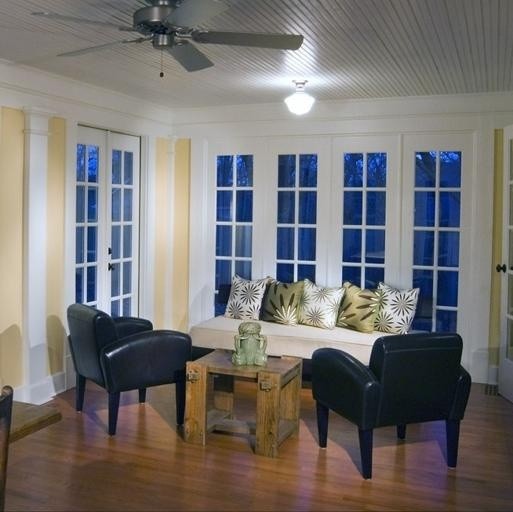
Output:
[[313, 333, 471, 479], [67, 304, 191, 436]]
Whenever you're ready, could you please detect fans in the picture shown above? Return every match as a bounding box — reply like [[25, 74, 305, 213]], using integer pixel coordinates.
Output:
[[29, 0, 304, 77]]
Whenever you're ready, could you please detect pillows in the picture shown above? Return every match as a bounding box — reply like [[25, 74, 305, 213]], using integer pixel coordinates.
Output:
[[373, 281, 421, 335], [223, 274, 271, 321], [335, 282, 381, 334], [298, 278, 346, 329], [259, 277, 304, 327]]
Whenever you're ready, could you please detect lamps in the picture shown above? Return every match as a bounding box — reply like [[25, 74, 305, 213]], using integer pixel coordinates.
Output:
[[284, 79, 315, 115]]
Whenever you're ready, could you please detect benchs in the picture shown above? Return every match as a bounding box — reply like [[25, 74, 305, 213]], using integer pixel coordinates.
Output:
[[189, 315, 428, 368]]
